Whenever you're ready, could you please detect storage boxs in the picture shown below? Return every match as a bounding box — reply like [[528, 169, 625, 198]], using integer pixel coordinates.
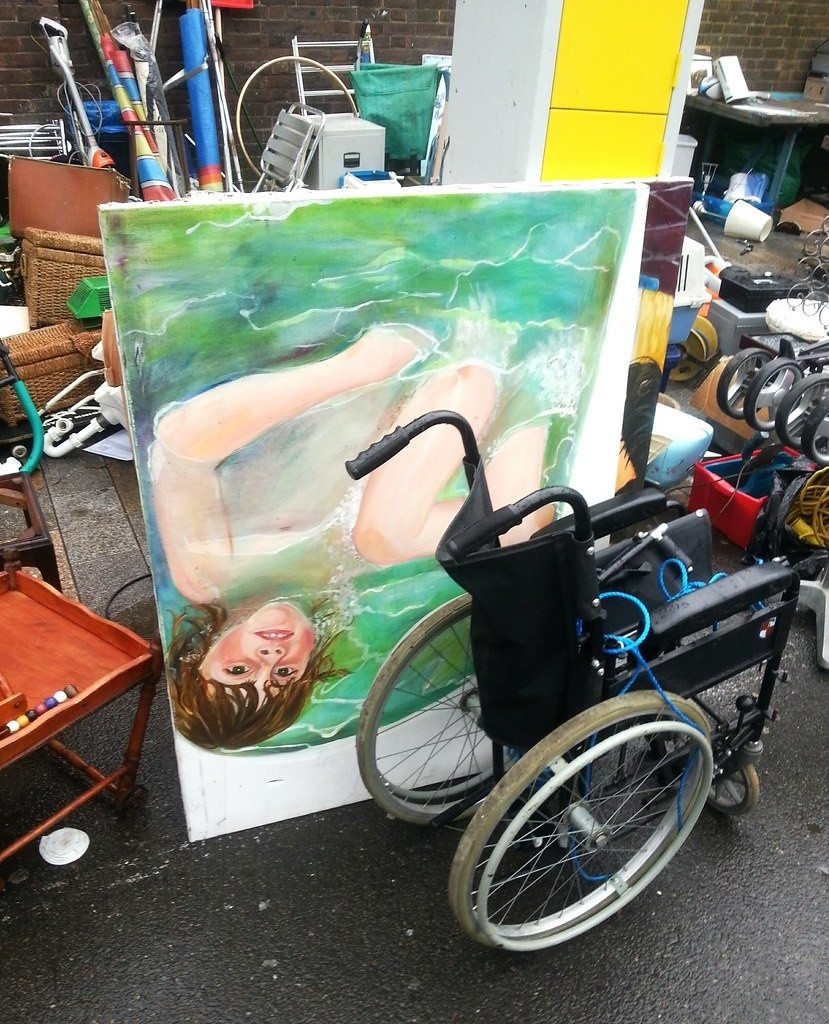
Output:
[[689, 49, 751, 104], [688, 442, 811, 550], [307, 114, 389, 193]]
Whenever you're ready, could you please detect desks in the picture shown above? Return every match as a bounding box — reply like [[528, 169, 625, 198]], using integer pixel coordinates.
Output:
[[687, 92, 828, 224]]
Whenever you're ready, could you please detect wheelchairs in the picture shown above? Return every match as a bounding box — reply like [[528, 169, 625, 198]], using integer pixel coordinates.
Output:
[[348, 408, 798, 950]]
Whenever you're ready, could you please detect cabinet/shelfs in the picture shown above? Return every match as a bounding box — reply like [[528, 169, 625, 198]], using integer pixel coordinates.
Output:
[[0, 568, 162, 877]]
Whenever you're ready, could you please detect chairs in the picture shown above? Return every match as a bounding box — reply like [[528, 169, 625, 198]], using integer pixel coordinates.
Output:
[[237, 101, 326, 196]]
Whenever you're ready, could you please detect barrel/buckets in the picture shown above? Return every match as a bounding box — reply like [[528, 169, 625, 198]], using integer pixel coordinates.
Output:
[[723, 199, 774, 242]]
